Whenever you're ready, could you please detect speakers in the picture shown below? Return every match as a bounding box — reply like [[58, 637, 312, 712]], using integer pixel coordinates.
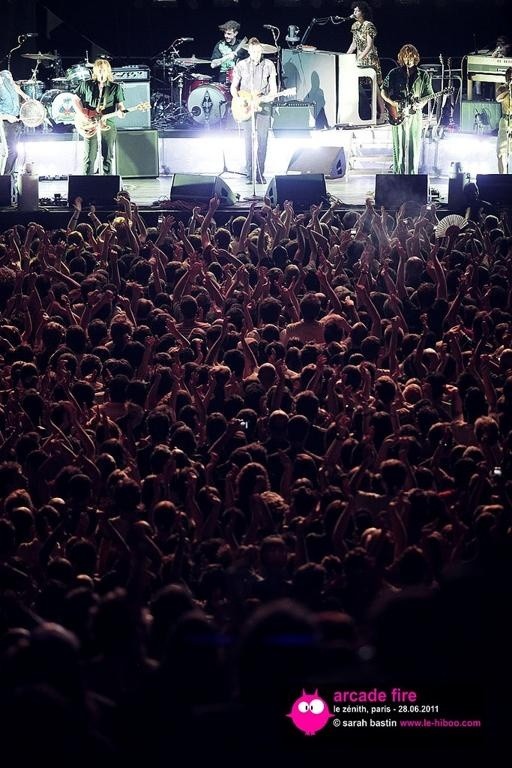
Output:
[[264, 173, 326, 210], [68, 175, 124, 208], [270, 105, 316, 130], [476, 174, 512, 207], [0, 175, 19, 207], [375, 173, 432, 207], [169, 173, 237, 206], [460, 100, 502, 136], [284, 146, 346, 179], [115, 130, 159, 178], [115, 81, 152, 130]]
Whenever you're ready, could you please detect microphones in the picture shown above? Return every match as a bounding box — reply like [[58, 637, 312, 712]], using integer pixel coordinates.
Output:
[[178, 38, 194, 41], [263, 25, 275, 29], [345, 14, 355, 20], [22, 33, 38, 38]]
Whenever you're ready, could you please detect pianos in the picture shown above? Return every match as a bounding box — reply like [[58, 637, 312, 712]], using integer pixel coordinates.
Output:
[[281, 45, 377, 128]]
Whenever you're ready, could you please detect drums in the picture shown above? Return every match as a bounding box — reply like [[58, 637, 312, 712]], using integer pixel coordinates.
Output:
[[16, 79, 44, 100], [226, 67, 234, 84], [39, 89, 83, 129], [182, 73, 213, 104], [186, 82, 231, 126], [20, 100, 45, 127], [68, 65, 92, 87], [50, 78, 71, 93]]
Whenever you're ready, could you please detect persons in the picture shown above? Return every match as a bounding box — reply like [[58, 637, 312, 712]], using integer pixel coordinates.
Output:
[[0, 1, 512, 766]]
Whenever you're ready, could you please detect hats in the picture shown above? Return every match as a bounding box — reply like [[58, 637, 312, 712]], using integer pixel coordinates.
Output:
[[218, 21, 240, 30]]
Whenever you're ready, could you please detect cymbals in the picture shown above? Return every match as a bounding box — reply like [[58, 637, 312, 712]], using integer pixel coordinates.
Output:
[[243, 42, 278, 54], [175, 57, 211, 63], [21, 54, 53, 60]]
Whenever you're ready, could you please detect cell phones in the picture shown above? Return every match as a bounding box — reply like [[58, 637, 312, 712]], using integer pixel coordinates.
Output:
[[493, 466, 502, 476], [240, 421, 249, 429]]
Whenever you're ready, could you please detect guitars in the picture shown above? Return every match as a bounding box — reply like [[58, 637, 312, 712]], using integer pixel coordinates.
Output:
[[75, 101, 151, 138], [384, 87, 455, 125], [231, 87, 298, 123]]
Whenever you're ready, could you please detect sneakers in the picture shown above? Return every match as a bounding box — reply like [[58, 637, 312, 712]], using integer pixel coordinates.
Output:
[[377, 109, 387, 124], [247, 176, 266, 184]]
